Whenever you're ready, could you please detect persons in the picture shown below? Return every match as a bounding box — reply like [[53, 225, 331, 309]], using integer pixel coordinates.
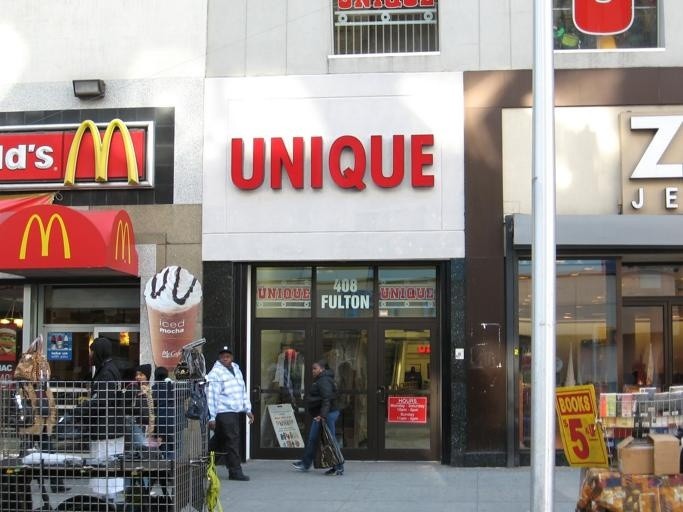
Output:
[[204, 344, 255, 480], [292, 359, 345, 477], [121, 361, 156, 433], [148, 363, 183, 453], [404, 364, 422, 389], [70, 338, 124, 441]]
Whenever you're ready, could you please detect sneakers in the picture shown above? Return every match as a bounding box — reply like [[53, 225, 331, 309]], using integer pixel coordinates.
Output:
[[323, 468, 343, 476], [290, 460, 312, 471], [228, 466, 251, 481], [50, 486, 71, 495]]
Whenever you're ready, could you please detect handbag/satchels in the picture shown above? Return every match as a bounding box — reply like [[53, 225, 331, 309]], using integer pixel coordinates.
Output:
[[36, 397, 50, 415], [184, 380, 211, 421], [13, 334, 52, 383], [55, 406, 88, 440], [16, 385, 34, 427], [206, 452, 224, 511], [174, 339, 207, 380], [313, 418, 344, 470]]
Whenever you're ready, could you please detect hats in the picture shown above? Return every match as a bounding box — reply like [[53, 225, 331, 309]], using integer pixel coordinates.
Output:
[[217, 343, 233, 354], [134, 363, 152, 381]]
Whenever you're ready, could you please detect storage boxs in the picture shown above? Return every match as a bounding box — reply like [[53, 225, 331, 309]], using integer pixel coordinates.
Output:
[[615, 431, 682, 476]]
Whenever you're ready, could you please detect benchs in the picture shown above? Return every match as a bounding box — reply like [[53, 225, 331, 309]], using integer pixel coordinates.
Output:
[[0, 453, 176, 511]]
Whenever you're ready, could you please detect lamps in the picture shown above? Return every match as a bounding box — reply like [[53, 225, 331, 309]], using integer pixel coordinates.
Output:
[[0, 296, 24, 330]]
